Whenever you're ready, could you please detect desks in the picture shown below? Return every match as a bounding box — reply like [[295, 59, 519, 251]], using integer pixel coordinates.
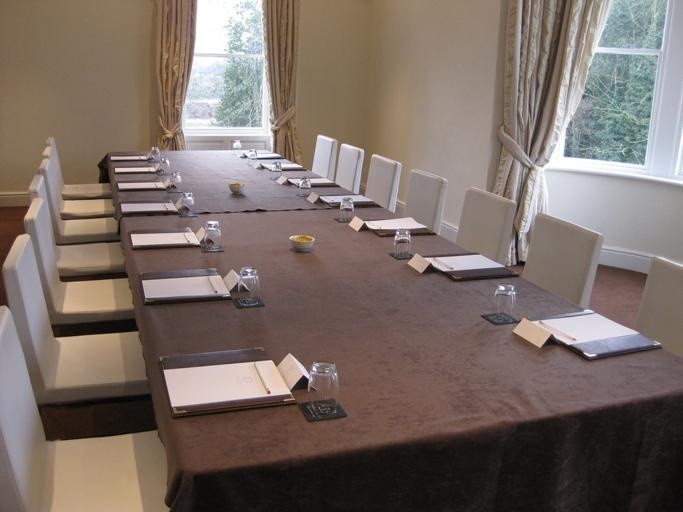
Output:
[[91, 146, 682, 511]]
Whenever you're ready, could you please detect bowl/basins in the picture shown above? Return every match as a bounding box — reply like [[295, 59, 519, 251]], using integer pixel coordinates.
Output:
[[228, 182, 246, 195], [288, 234, 315, 253]]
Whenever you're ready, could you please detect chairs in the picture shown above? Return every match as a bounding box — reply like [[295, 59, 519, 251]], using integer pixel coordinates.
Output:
[[311, 134, 363, 194], [457, 186, 681, 357], [0, 134, 171, 511], [362, 155, 447, 232]]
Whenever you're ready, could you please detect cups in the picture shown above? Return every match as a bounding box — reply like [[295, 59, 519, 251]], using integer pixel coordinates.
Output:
[[150, 146, 160, 164], [180, 190, 195, 217], [271, 161, 281, 180], [201, 220, 222, 252], [392, 229, 411, 258], [168, 172, 182, 191], [307, 361, 339, 415], [339, 197, 354, 221], [494, 283, 515, 321], [299, 176, 311, 197], [237, 266, 260, 306], [247, 149, 257, 166], [157, 156, 171, 176], [231, 140, 241, 156]]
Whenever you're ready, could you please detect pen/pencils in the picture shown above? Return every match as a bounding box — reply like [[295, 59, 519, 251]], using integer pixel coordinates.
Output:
[[164, 203, 168, 209], [154, 182, 158, 188], [367, 220, 383, 228], [208, 277, 217, 293], [539, 320, 575, 341], [432, 258, 455, 269], [184, 233, 190, 242], [254, 363, 271, 394], [323, 196, 333, 201]]
